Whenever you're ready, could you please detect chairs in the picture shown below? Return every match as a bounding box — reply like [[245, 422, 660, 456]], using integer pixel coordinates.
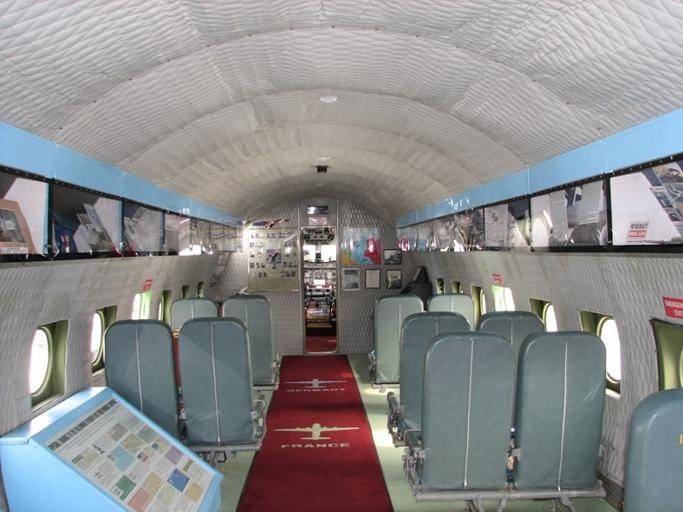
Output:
[[100, 293, 282, 467]]
